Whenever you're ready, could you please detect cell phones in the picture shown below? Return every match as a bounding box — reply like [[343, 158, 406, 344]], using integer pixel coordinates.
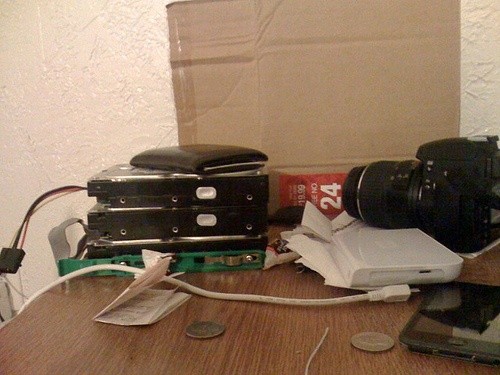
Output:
[[399, 282, 499, 365]]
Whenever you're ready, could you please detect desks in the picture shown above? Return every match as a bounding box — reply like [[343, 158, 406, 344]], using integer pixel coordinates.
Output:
[[0, 225, 499, 375]]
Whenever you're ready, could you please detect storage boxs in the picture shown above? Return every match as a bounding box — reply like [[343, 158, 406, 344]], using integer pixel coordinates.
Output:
[[166, 0, 465, 226]]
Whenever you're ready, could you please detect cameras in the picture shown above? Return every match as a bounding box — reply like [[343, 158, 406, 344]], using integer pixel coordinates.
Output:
[[341, 136, 500, 252]]
